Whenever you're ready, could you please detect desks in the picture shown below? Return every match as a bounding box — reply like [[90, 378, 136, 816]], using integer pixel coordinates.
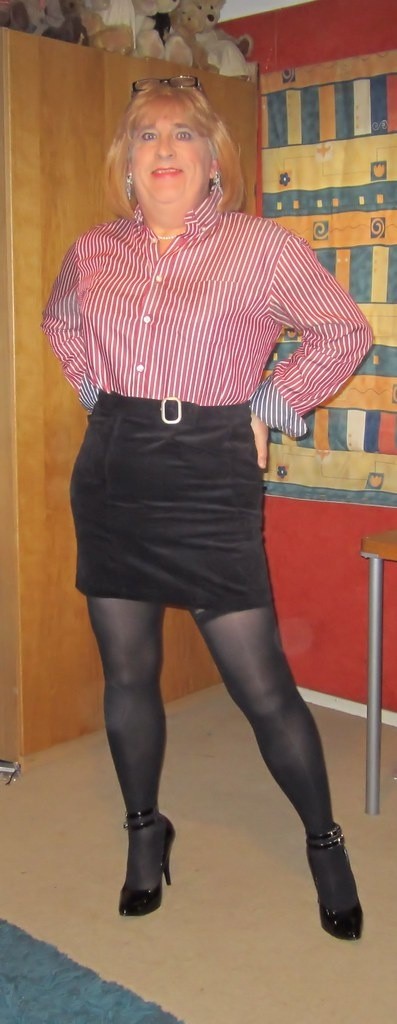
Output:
[[360, 522, 397, 816]]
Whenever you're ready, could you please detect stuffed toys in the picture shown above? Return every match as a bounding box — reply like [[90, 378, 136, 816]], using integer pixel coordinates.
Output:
[[0, 0, 250, 78]]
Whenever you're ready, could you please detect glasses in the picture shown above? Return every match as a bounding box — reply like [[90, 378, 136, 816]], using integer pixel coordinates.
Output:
[[131, 75, 210, 99]]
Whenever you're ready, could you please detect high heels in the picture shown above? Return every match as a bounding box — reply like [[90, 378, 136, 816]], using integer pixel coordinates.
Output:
[[117, 806, 177, 918], [303, 822, 364, 942]]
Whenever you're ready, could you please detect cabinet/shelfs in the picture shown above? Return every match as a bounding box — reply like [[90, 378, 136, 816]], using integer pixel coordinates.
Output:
[[1, 26, 261, 779]]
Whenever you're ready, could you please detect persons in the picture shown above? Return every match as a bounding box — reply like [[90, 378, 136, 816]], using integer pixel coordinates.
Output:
[[41, 76, 374, 942]]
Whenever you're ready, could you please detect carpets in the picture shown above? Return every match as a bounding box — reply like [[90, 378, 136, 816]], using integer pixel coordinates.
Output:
[[0, 916, 186, 1024]]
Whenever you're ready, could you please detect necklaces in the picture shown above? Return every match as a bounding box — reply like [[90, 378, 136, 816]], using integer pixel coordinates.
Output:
[[157, 235, 179, 240]]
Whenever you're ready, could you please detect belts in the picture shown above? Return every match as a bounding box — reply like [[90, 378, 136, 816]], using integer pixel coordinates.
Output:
[[87, 389, 254, 431]]
